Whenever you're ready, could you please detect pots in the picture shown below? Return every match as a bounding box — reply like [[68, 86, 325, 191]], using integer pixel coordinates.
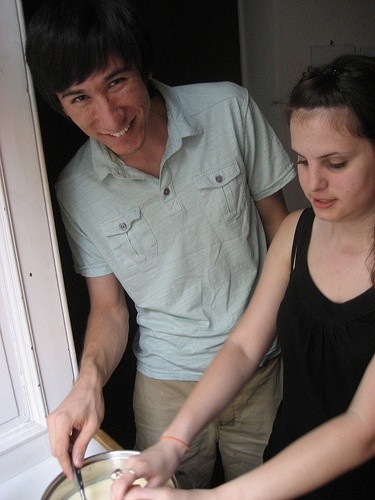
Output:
[[41, 450, 178, 500]]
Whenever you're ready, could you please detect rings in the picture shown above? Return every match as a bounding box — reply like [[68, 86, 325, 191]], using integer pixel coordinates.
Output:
[[127, 469, 138, 479]]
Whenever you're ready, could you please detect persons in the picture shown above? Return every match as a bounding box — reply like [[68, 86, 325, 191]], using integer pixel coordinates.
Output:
[[111, 54, 375, 500], [25, 0, 299, 489]]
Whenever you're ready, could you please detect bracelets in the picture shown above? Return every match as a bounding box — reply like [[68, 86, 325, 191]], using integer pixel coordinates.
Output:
[[159, 436, 190, 450]]
